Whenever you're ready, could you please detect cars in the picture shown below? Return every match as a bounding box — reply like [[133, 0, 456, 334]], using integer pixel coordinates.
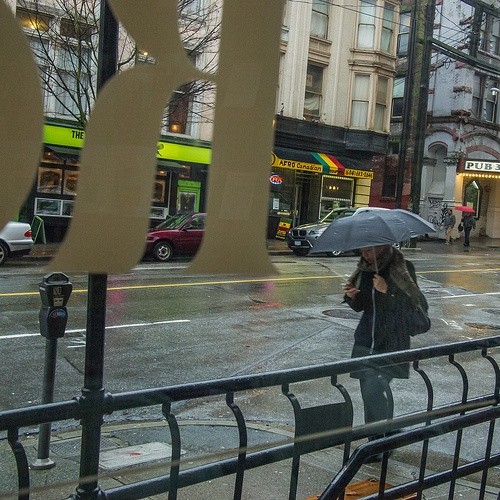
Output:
[[0, 221, 34, 269], [146, 213, 206, 261]]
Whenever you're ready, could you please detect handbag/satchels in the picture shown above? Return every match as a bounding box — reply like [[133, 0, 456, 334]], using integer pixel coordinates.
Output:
[[458, 223, 463, 232], [401, 300, 431, 335]]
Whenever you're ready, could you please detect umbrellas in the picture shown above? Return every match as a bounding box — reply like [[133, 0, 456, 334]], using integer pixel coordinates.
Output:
[[455, 206, 475, 216], [308, 209, 437, 276]]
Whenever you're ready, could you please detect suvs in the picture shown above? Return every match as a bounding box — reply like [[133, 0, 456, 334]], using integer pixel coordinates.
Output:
[[285, 208, 402, 257]]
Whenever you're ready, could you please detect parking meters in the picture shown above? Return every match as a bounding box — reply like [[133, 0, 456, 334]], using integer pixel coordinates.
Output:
[[30, 270, 73, 471]]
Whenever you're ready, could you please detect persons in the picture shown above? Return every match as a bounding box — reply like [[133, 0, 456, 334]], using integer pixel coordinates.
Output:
[[458, 211, 476, 247], [439, 208, 456, 245], [342, 245, 429, 464]]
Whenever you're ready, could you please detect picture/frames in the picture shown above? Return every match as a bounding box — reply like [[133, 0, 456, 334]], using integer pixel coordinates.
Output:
[[34, 152, 168, 220]]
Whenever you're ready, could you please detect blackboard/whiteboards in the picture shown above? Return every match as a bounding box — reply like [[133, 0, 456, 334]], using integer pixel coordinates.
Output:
[[30, 216, 46, 244]]
